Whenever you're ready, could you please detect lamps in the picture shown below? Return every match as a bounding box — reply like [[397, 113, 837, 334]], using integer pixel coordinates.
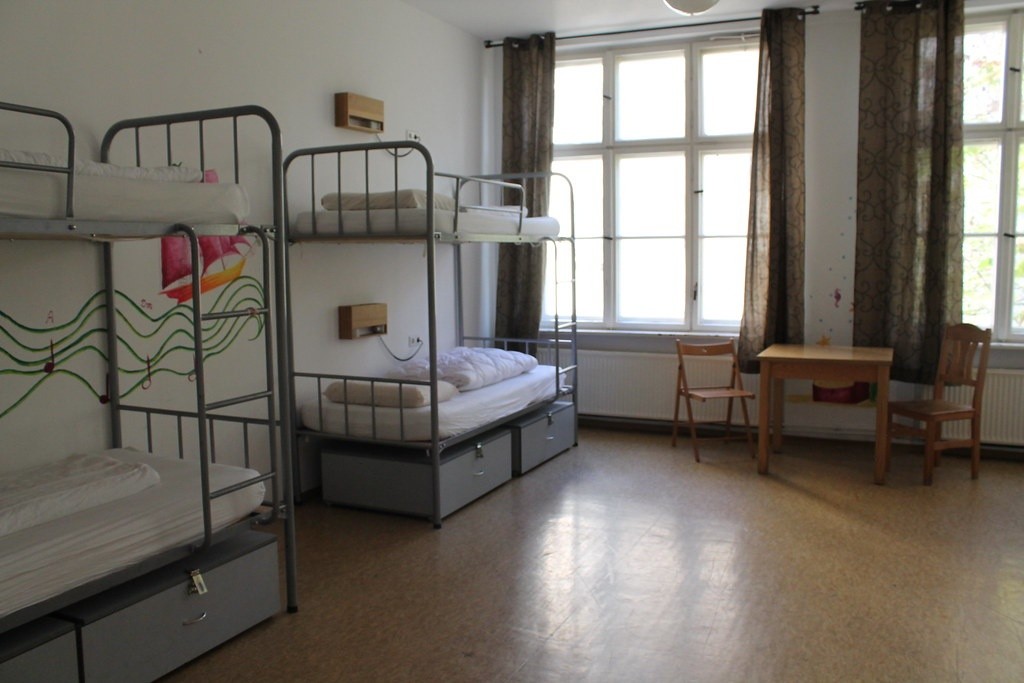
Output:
[[665, 0, 718, 15]]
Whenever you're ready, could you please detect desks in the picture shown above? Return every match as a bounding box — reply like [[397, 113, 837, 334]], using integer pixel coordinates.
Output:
[[756, 344, 894, 485]]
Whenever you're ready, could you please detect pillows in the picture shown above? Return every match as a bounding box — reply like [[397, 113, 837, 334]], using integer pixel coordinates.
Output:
[[321, 188, 456, 211], [323, 378, 460, 408]]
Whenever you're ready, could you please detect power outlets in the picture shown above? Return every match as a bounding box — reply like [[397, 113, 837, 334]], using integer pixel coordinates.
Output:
[[407, 131, 419, 142], [408, 335, 425, 347]]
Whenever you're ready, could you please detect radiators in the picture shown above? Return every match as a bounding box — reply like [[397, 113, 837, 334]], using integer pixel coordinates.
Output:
[[921, 366, 1024, 446], [535, 347, 760, 427]]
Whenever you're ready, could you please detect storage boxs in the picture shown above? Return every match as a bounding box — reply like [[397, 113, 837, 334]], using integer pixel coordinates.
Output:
[[508, 401, 575, 476], [57, 529, 283, 683], [0, 616, 80, 683], [320, 427, 514, 519]]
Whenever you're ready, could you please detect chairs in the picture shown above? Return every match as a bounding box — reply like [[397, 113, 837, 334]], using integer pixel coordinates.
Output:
[[671, 338, 755, 463], [887, 323, 991, 486]]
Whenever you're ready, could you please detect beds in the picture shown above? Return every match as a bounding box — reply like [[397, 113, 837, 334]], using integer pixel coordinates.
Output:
[[1, 147, 250, 229], [301, 346, 567, 442], [0, 446, 267, 620], [295, 188, 560, 236]]
